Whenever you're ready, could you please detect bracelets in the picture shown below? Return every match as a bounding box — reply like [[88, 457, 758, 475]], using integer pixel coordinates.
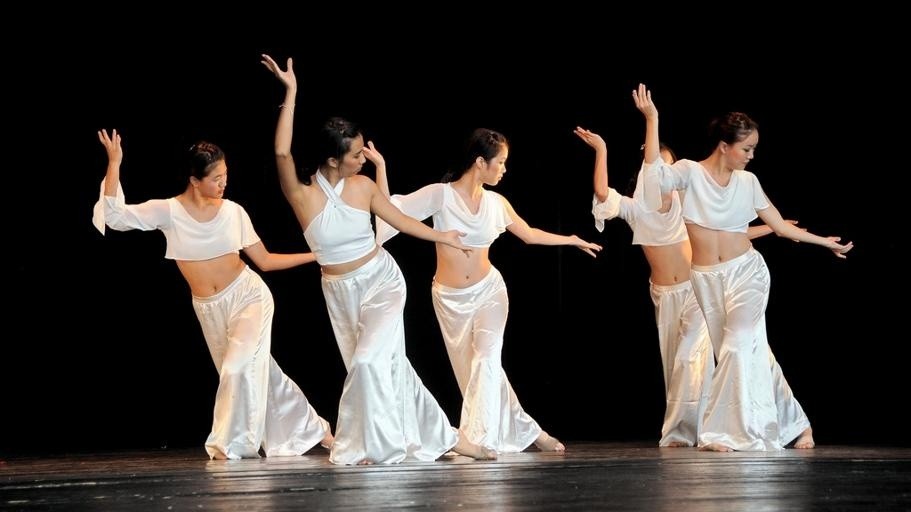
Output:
[[279, 103, 296, 109]]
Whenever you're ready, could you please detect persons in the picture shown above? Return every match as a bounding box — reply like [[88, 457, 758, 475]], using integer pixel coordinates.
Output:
[[363, 122, 603, 453], [91, 127, 336, 460], [571, 125, 809, 448], [261, 55, 497, 465], [630, 82, 855, 453]]
